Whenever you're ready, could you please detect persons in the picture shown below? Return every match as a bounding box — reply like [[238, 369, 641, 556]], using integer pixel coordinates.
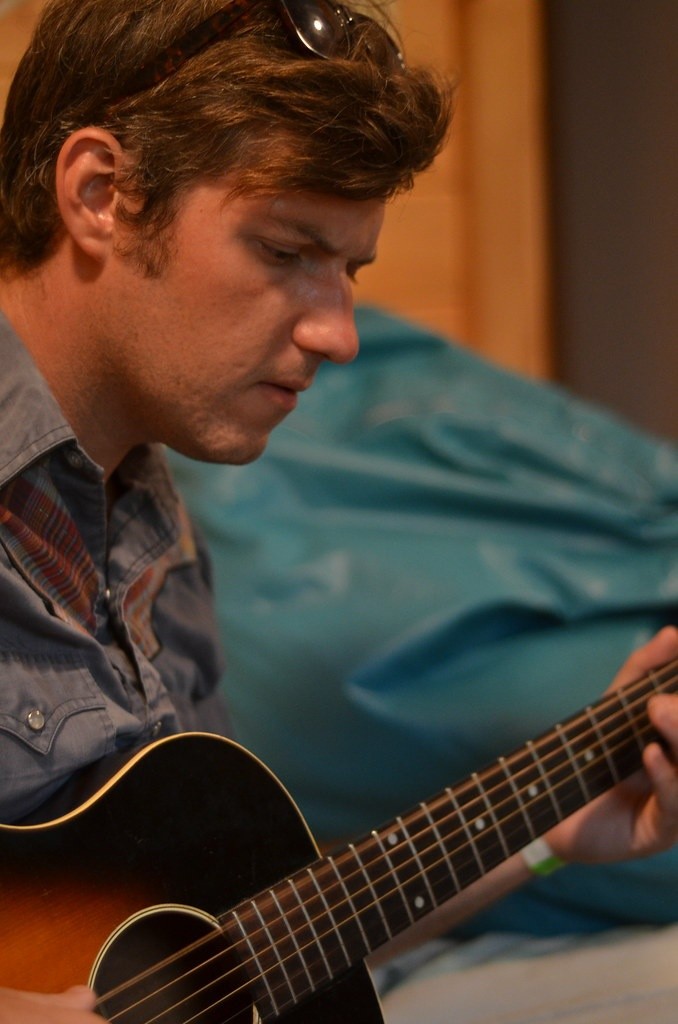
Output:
[[3, 2, 676, 1024]]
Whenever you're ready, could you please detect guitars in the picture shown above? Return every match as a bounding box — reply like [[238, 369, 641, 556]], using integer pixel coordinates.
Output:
[[0, 653, 678, 1023]]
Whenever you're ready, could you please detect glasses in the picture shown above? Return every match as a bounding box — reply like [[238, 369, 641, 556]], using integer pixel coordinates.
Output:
[[68, 0, 406, 128]]
